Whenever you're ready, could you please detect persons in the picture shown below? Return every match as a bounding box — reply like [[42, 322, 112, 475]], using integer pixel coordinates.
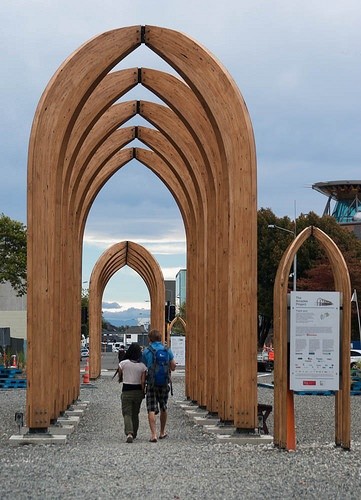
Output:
[[141, 329, 176, 442], [262, 335, 274, 352], [118, 342, 146, 443], [118, 345, 126, 363]]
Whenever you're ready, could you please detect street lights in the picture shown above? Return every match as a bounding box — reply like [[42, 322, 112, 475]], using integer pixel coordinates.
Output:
[[176, 296, 182, 334], [267, 199, 297, 293], [82, 281, 88, 333]]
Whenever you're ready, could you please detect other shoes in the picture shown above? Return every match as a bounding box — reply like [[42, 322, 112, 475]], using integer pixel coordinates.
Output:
[[126, 434, 134, 442]]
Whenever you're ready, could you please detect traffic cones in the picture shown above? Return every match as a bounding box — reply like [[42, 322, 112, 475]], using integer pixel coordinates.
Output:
[[80, 359, 91, 385], [11, 355, 17, 366]]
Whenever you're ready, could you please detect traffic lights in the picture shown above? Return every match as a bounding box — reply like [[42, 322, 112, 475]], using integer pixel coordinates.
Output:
[[168, 305, 176, 322]]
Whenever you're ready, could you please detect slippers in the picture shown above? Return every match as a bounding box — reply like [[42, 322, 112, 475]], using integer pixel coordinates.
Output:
[[149, 438, 157, 442], [160, 432, 167, 439]]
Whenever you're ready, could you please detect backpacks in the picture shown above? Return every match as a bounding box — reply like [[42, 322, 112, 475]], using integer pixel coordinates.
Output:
[[148, 346, 171, 385]]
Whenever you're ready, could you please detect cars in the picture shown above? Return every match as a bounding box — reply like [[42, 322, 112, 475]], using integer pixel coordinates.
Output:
[[112, 347, 126, 353], [350, 349, 361, 370], [81, 349, 89, 357]]
